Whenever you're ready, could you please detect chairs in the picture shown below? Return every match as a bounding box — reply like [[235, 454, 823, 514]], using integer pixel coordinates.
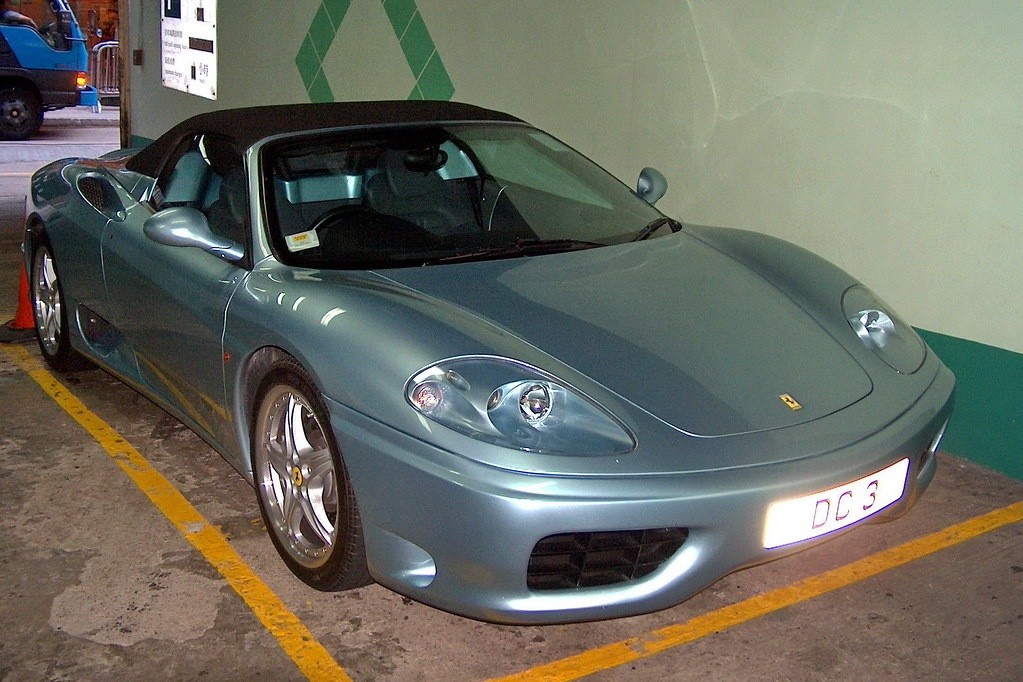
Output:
[[364, 150, 473, 239], [206, 167, 310, 248]]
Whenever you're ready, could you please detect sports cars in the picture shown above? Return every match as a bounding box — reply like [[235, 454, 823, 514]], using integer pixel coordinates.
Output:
[[21, 101, 958, 627]]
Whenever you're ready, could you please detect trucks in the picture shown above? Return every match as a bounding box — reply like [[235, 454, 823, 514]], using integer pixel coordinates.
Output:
[[0, 0, 103, 142]]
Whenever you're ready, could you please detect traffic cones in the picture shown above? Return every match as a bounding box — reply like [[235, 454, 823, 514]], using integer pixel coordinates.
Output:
[[0, 194, 36, 344]]
